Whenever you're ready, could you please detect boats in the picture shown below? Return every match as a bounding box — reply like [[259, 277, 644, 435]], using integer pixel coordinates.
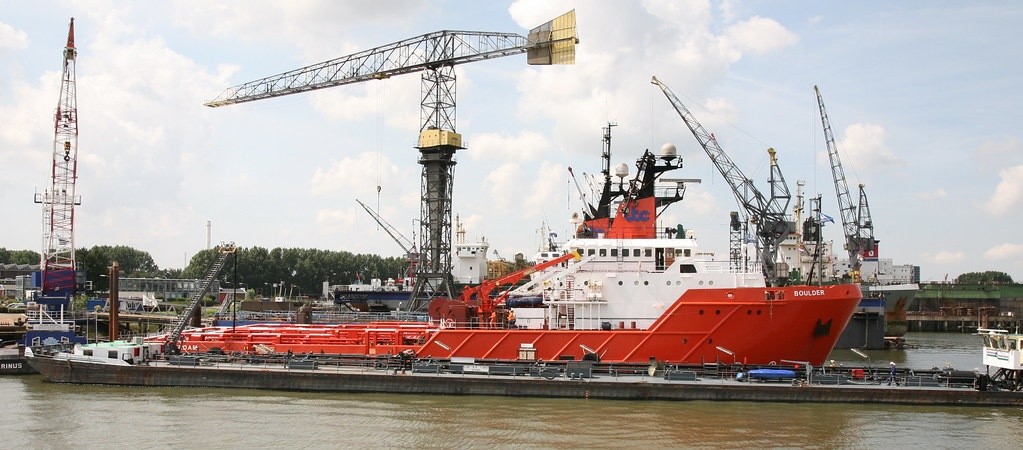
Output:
[[0, 75, 1023, 406]]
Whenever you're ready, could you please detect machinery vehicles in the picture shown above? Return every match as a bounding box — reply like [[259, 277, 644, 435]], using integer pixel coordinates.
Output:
[[429, 251, 581, 330]]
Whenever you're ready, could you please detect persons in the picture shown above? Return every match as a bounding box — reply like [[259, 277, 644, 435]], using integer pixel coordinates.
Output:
[[503, 311, 517, 327], [488, 310, 497, 327], [211, 314, 220, 326]]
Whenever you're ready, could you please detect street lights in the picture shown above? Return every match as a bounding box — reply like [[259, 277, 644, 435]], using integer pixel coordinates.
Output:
[[264, 282, 272, 302], [239, 283, 248, 292]]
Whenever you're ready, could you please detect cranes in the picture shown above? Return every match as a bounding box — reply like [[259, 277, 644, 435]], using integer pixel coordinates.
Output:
[[34, 15, 82, 314], [204, 8, 580, 324]]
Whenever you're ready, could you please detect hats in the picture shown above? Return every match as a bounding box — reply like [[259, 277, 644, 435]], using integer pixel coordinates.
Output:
[[890, 362, 896, 365]]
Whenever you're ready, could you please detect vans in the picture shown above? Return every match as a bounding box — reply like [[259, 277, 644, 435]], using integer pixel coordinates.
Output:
[[5, 302, 27, 310]]
